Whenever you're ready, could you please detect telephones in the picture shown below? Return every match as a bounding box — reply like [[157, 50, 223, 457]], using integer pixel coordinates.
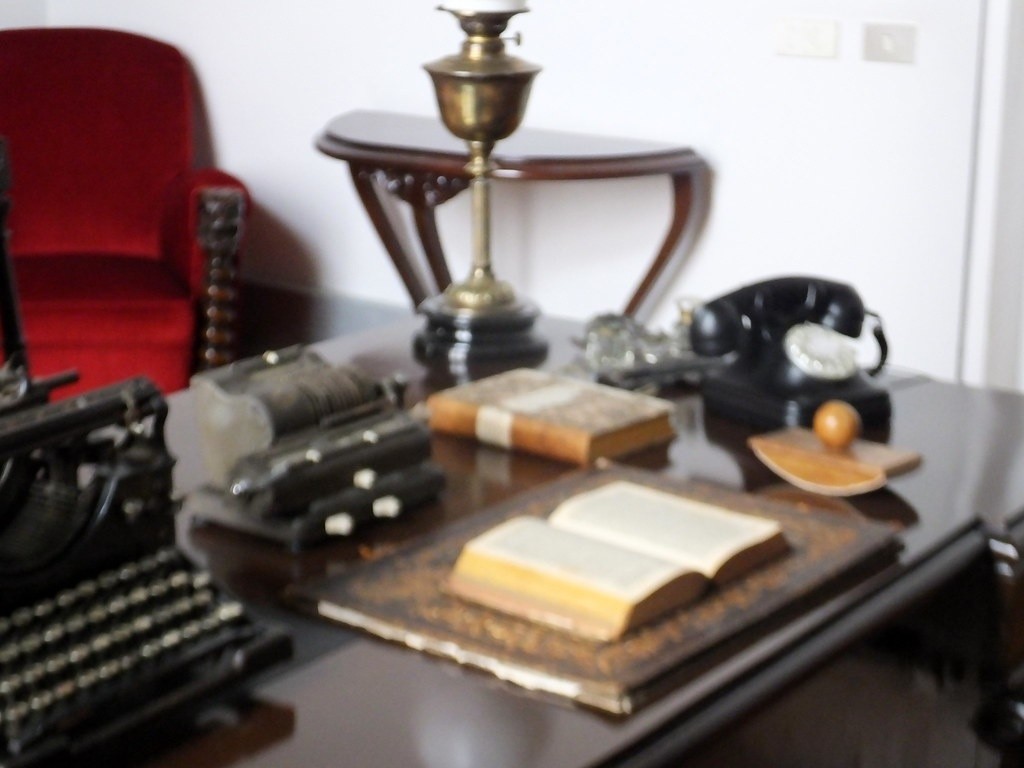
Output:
[[687, 274, 891, 432]]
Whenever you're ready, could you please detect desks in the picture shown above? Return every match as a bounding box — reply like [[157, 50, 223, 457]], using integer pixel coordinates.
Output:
[[0, 306, 1022, 768], [312, 108, 710, 322]]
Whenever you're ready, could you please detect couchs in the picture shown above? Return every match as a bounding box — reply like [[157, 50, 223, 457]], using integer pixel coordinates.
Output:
[[0, 26, 251, 404]]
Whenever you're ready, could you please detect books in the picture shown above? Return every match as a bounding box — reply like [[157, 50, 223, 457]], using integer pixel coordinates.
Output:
[[427, 365, 680, 463], [443, 480, 789, 643]]
